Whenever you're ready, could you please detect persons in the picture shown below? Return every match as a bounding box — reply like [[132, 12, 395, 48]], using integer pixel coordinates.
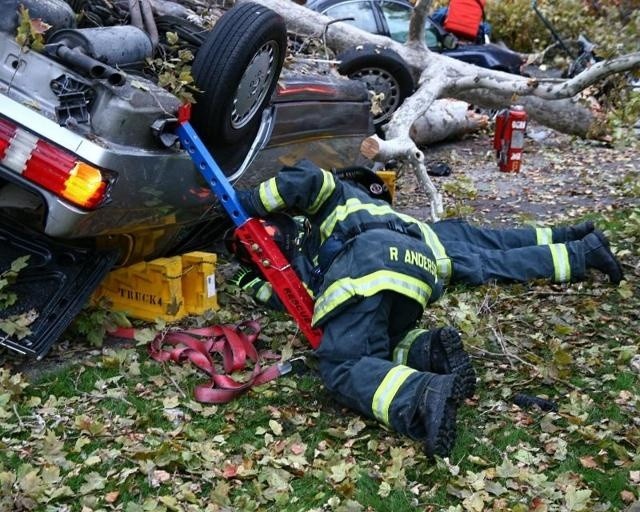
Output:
[[223, 207, 625, 311], [235, 165, 475, 458]]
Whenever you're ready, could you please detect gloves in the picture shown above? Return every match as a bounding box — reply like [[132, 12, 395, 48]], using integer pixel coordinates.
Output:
[[225, 264, 272, 304]]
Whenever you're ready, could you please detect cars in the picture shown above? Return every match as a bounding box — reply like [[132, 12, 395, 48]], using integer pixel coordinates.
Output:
[[0, 1, 414, 361], [298, 1, 529, 128]]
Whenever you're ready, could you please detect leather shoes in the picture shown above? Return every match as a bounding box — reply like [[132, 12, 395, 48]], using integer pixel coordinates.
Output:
[[419, 374, 463, 461], [571, 221, 595, 238], [582, 231, 624, 285], [430, 327, 476, 399]]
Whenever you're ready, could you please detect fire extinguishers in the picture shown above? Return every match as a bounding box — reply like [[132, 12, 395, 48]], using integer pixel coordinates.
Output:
[[493, 105, 528, 172]]
